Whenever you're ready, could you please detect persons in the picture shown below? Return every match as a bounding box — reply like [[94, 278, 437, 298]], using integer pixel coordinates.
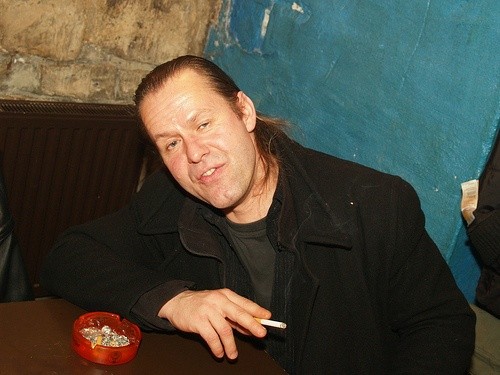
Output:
[[36, 55, 477, 375]]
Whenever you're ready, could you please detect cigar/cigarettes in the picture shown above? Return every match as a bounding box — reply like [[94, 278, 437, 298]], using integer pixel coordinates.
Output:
[[96, 335, 102, 345], [254, 317, 287, 329]]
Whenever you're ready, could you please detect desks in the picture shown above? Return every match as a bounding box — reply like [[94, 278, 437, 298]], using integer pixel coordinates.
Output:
[[1, 296, 290, 375]]
